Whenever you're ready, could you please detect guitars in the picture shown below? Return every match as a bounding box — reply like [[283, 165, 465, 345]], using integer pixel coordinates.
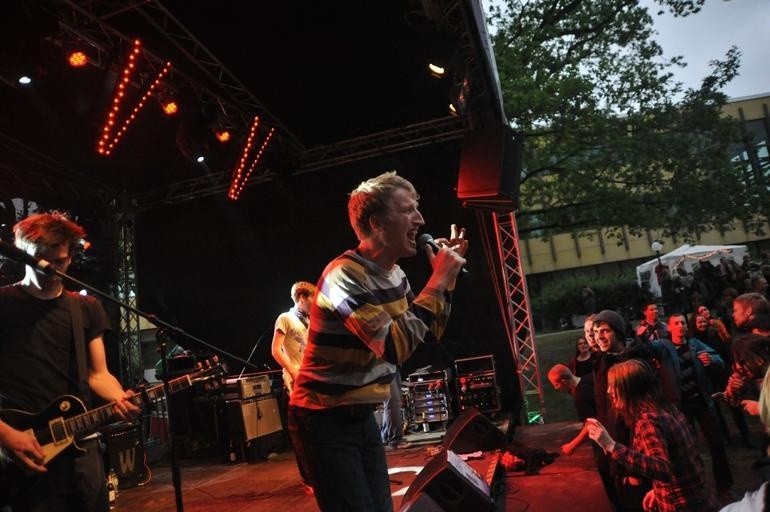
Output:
[[0, 355, 230, 479]]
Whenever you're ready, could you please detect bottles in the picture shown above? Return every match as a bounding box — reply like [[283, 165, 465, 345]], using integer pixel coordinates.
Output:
[[107, 476, 115, 510], [108, 466, 119, 497]]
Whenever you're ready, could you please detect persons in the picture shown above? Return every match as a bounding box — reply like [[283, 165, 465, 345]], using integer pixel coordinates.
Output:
[[0, 210, 138, 511], [632, 249, 768, 320], [285, 172, 470, 511], [547, 285, 769, 510], [270, 281, 321, 496], [381, 369, 413, 450]]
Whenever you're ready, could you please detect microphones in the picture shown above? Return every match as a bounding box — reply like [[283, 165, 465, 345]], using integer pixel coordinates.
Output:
[[0, 239, 56, 277], [418, 233, 470, 280]]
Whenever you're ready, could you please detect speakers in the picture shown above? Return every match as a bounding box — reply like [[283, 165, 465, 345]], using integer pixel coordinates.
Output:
[[457, 124, 521, 205], [100, 422, 145, 489], [399, 446, 492, 512], [398, 491, 445, 512], [441, 403, 504, 456]]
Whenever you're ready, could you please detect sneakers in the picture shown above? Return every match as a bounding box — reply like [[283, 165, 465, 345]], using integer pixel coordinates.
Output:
[[382, 440, 412, 448]]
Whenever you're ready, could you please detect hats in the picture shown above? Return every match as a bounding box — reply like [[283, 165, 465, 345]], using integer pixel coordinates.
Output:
[[591, 310, 627, 336]]
